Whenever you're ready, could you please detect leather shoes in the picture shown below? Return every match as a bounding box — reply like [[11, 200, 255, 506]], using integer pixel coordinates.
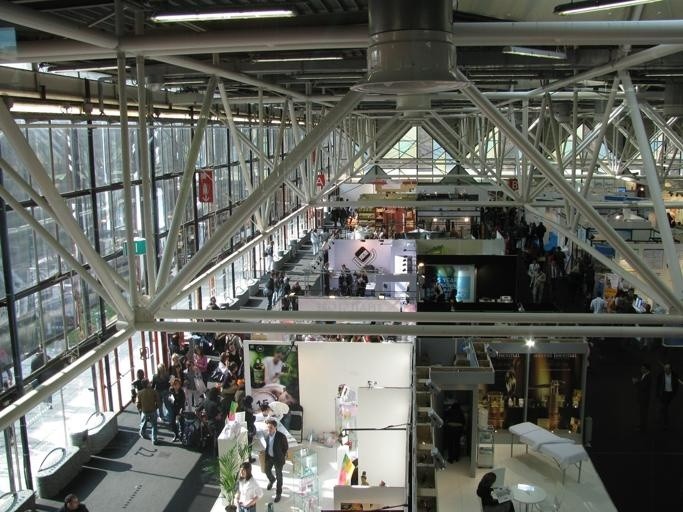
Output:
[[173, 434, 178, 442]]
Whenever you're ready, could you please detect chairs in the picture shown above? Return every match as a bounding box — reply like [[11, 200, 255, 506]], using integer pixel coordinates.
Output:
[[477, 468, 565, 512]]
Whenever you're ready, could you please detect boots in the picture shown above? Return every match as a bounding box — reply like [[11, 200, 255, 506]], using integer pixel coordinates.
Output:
[[249, 456, 256, 463]]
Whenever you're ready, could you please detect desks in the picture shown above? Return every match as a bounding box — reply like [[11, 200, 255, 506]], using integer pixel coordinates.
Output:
[[509, 422, 587, 485]]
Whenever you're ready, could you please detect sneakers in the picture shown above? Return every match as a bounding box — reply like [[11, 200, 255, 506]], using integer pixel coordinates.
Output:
[[139, 429, 145, 439], [267, 478, 276, 490], [274, 494, 281, 502], [154, 439, 160, 444]]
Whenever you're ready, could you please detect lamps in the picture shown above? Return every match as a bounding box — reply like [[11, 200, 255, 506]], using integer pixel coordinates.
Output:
[[425, 379, 447, 471]]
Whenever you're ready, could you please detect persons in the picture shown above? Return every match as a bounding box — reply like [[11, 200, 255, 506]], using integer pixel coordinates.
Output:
[[257, 350, 285, 384], [0, 347, 15, 447], [58, 493, 90, 512], [311, 229, 328, 255], [424, 206, 656, 450], [323, 263, 368, 297], [262, 269, 302, 311], [332, 198, 351, 226], [655, 358, 681, 432], [476, 471, 516, 511], [263, 381, 298, 405], [262, 419, 288, 501], [439, 402, 465, 463], [236, 461, 263, 511], [132, 297, 256, 462], [29, 349, 52, 410]]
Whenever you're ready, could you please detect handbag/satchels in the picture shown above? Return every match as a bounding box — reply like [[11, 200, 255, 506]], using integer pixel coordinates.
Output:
[[248, 425, 256, 437], [194, 378, 206, 394], [262, 285, 273, 297]]
